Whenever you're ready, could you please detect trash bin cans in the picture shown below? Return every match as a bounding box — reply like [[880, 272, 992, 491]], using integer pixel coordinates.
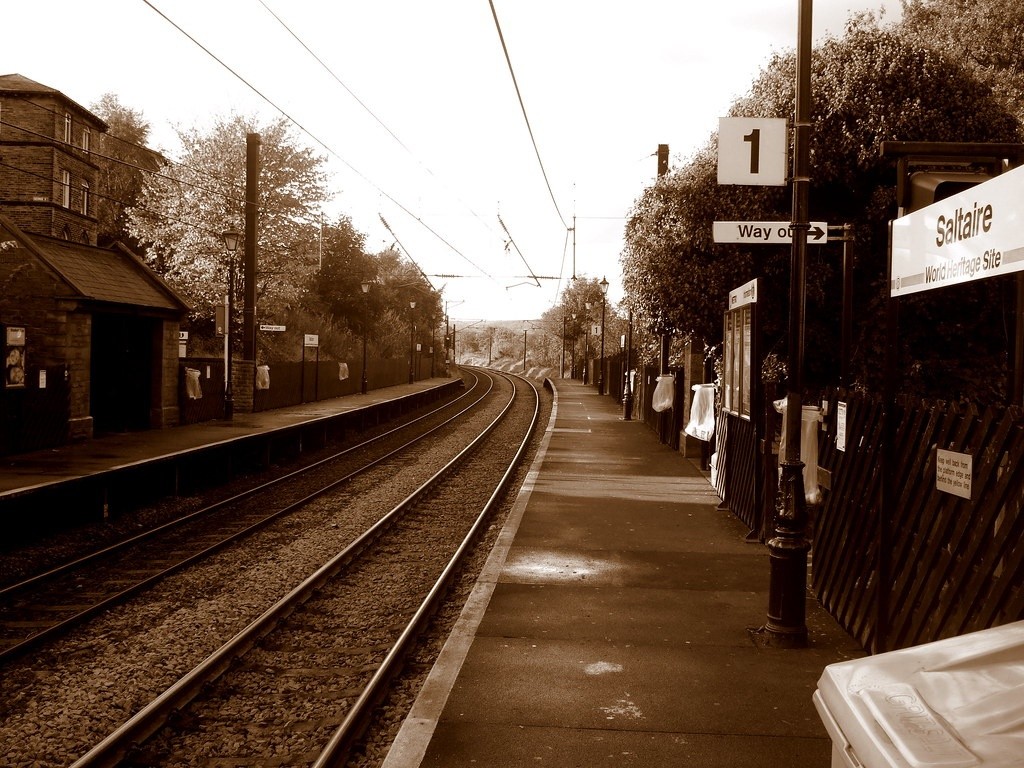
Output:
[[813, 620, 1024, 768]]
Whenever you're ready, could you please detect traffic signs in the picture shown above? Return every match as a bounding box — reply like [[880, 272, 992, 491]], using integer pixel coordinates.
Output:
[[711, 219, 828, 245]]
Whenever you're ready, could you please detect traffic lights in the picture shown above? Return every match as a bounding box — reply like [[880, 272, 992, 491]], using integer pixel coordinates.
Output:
[[443, 335, 452, 350]]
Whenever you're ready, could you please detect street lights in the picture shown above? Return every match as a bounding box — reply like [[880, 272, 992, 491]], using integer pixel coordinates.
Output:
[[599, 275, 610, 395], [360, 279, 371, 393], [522, 329, 528, 371], [408, 296, 417, 385], [430, 311, 437, 379], [560, 315, 567, 380], [583, 299, 593, 386], [570, 309, 579, 380], [220, 220, 244, 419]]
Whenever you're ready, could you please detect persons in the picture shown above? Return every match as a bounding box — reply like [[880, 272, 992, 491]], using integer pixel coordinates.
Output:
[[7, 345, 24, 384]]
